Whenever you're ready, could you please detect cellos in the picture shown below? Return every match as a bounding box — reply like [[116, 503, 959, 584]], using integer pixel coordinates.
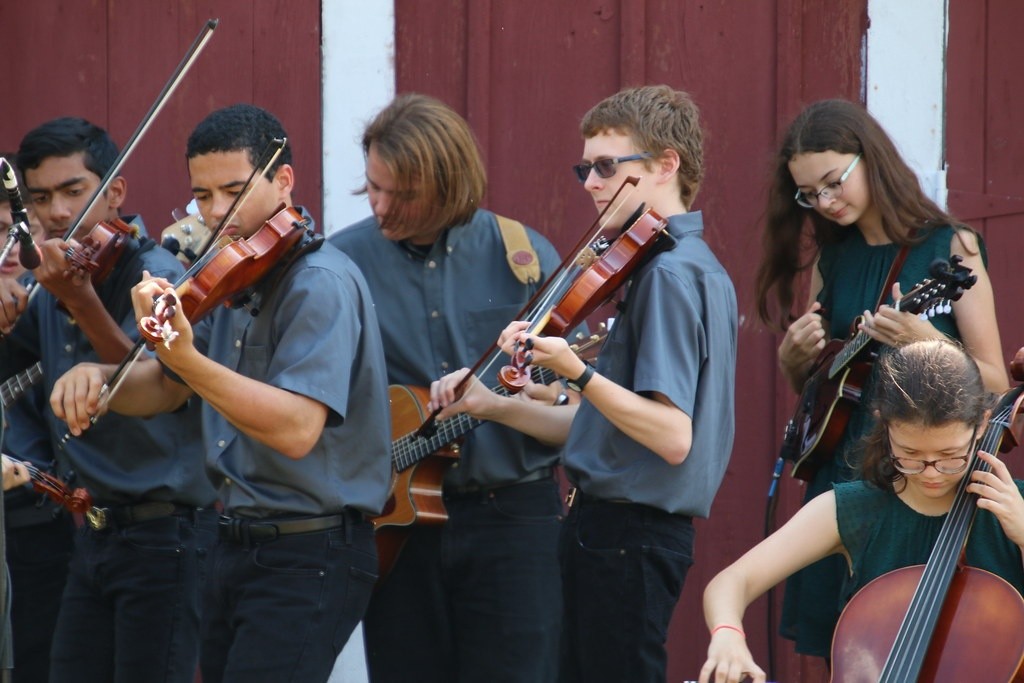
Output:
[[828, 342, 1024, 683]]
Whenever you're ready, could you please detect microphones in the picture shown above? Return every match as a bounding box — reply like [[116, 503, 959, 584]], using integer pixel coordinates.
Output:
[[930, 259, 952, 281], [160, 236, 181, 256], [2, 161, 42, 269]]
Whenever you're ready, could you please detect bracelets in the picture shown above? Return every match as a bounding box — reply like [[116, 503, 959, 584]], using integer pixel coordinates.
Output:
[[710, 624, 746, 638]]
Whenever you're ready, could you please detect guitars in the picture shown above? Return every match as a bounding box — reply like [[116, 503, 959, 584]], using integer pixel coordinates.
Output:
[[791, 252, 979, 476], [372, 316, 618, 581]]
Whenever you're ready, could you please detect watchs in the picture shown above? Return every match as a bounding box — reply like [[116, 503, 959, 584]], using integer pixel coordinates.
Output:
[[564, 359, 597, 393]]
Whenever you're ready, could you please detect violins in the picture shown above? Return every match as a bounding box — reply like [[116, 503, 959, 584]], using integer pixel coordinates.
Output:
[[495, 208, 669, 394], [137, 205, 308, 341], [0, 449, 114, 530], [64, 218, 134, 289]]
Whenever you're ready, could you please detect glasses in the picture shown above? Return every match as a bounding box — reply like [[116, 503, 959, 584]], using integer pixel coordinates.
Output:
[[795, 152, 866, 208], [573, 149, 658, 184], [887, 424, 980, 476]]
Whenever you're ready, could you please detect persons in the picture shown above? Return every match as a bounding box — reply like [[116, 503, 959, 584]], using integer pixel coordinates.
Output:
[[427, 86, 738, 683], [702, 340, 1024, 683], [754, 100, 1009, 656], [326, 92, 591, 683], [0, 104, 392, 683]]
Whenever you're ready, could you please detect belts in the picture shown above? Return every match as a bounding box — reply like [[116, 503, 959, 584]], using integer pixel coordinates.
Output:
[[563, 488, 694, 524], [84, 500, 209, 532], [444, 465, 558, 495], [4, 501, 64, 528], [217, 508, 368, 542]]
